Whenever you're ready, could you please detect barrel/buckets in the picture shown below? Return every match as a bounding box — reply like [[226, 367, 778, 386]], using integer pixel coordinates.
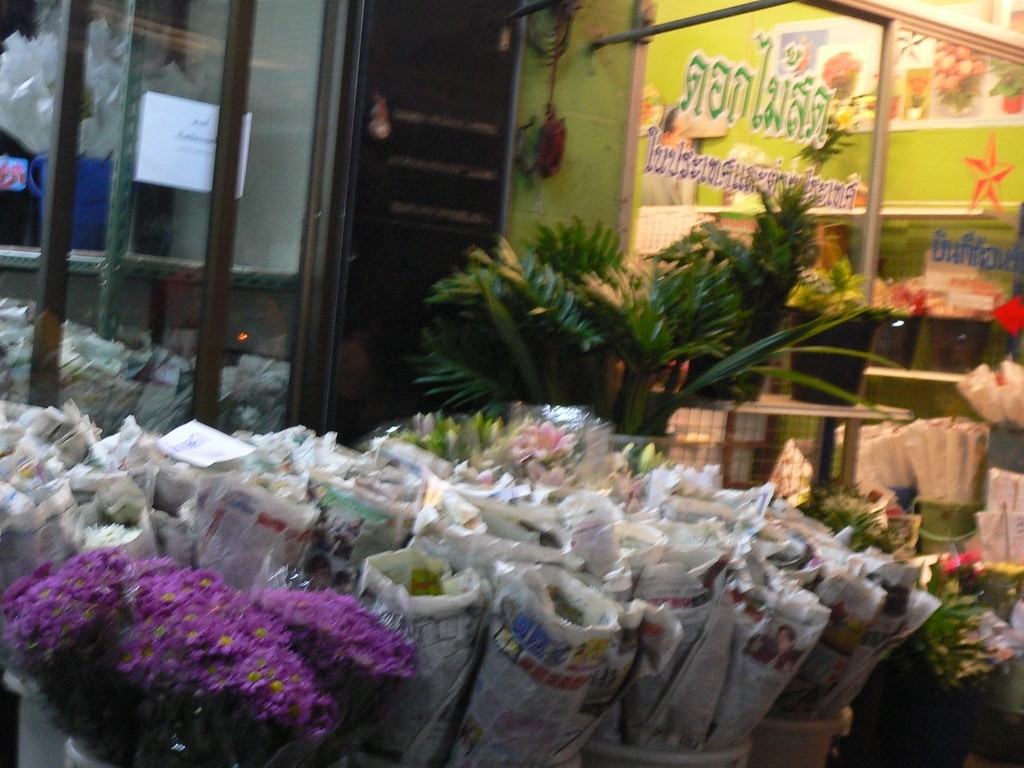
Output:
[[28, 152, 139, 252], [582, 740, 751, 768], [912, 497, 982, 554], [752, 707, 852, 768]]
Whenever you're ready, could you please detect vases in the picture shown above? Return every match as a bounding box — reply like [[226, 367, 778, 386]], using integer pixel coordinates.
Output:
[[25, 153, 112, 250]]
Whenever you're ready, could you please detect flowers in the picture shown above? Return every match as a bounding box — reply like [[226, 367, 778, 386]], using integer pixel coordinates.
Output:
[[0, 13, 1024, 768]]
